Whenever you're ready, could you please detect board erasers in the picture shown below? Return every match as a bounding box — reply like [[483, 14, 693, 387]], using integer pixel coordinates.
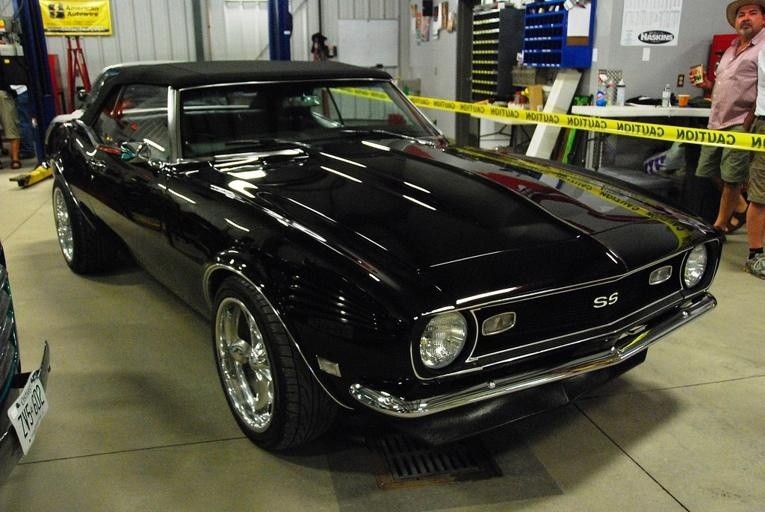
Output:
[[376, 65, 383, 68]]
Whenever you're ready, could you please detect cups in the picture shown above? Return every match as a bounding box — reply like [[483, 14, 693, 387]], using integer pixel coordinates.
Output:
[[678, 95, 689, 106]]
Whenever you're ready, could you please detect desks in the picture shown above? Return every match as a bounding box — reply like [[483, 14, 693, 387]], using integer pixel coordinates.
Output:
[[571, 104, 713, 172]]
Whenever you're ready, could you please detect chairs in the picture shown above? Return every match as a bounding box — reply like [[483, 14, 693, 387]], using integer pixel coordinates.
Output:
[[175, 105, 318, 154]]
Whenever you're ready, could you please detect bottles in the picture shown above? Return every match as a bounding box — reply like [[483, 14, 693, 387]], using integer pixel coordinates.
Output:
[[606, 80, 616, 105], [616, 79, 626, 106], [661, 84, 672, 107]]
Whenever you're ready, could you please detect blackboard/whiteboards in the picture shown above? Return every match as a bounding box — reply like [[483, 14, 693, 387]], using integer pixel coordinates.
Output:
[[337, 18, 400, 69]]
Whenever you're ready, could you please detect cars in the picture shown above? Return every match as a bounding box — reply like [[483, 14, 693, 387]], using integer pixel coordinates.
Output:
[[0, 242, 52, 486], [42, 60, 727, 455]]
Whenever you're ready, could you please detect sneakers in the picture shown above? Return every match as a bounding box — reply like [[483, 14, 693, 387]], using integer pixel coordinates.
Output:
[[743, 252, 765, 281]]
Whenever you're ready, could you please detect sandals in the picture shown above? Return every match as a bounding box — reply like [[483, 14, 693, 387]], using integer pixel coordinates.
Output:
[[711, 204, 749, 243]]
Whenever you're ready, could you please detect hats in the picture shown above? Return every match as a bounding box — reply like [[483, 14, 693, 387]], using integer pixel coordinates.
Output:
[[725, 0, 765, 28]]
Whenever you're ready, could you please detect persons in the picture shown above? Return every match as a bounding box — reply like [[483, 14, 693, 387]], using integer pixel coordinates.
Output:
[[9, 85, 36, 158], [688, 0, 765, 241], [1, 87, 22, 170], [745, 41, 765, 280]]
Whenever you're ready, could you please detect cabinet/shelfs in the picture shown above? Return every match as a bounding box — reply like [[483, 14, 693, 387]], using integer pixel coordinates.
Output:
[[470, 0, 597, 105]]
